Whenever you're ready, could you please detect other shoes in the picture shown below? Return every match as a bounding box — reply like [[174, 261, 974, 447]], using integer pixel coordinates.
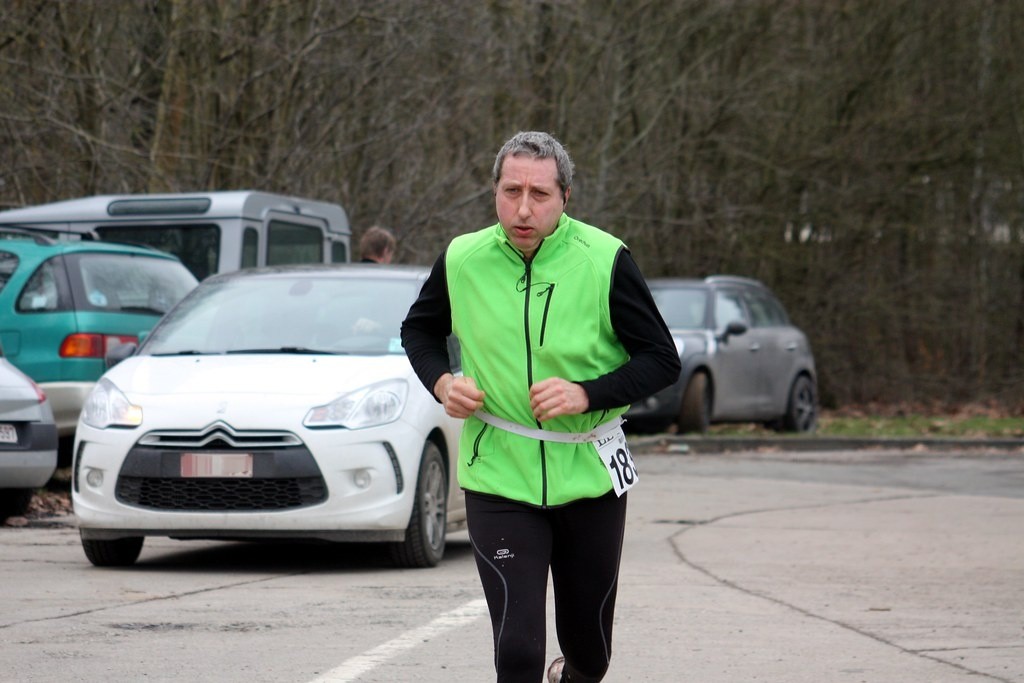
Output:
[[548, 657, 565, 683]]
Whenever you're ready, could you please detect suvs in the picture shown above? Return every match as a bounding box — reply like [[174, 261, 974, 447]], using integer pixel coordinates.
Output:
[[0, 222, 204, 471], [622, 275, 821, 436]]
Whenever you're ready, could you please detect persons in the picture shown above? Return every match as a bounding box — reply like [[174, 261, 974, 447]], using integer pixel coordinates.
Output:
[[360, 228, 396, 263], [398, 131, 683, 683]]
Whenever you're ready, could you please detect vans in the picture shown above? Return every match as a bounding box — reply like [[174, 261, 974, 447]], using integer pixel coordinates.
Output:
[[0, 189, 352, 314]]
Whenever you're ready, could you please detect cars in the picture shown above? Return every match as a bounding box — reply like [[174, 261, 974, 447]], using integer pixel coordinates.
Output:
[[1, 352, 61, 521], [70, 263, 472, 564]]
